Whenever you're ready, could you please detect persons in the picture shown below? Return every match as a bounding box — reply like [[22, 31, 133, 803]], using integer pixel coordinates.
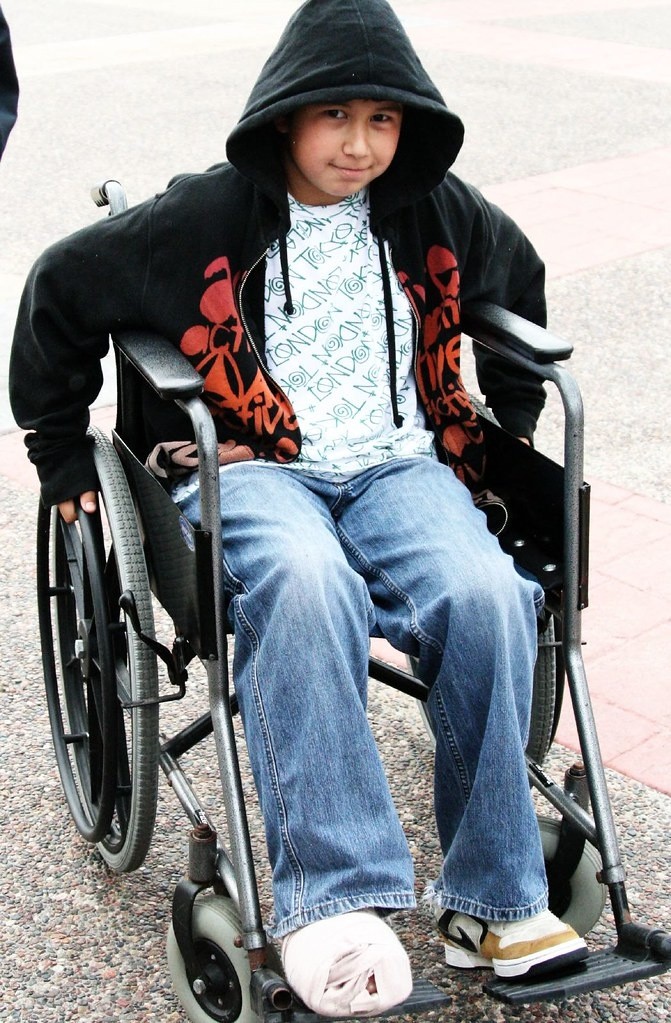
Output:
[[7, 1, 595, 1016]]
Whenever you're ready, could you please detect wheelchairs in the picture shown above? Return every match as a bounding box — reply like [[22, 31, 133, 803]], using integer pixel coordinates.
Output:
[[34, 178, 671, 1023]]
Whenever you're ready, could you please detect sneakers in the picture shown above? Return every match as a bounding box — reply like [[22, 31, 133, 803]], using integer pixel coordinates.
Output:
[[434, 904, 590, 980]]
